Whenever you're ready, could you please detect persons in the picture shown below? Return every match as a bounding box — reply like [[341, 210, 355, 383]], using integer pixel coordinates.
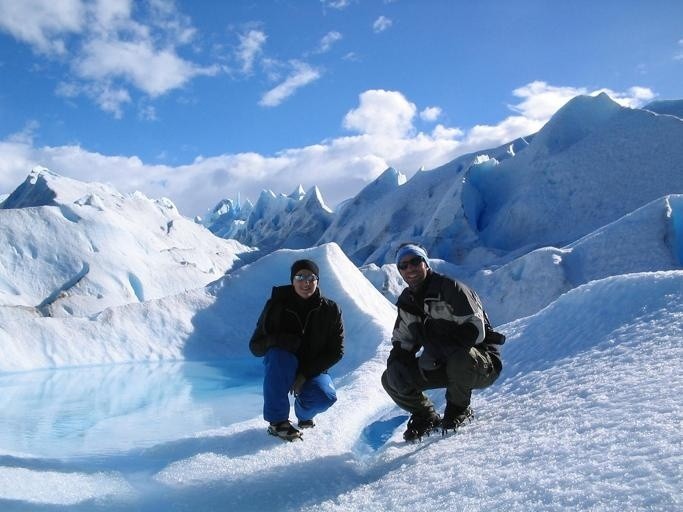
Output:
[[249, 259, 344, 440], [381, 242, 506, 441]]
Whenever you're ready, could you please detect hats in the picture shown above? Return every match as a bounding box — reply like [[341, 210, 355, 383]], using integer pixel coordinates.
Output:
[[291, 259, 319, 282]]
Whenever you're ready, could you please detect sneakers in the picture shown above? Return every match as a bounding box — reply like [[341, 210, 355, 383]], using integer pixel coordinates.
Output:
[[267, 420, 300, 440], [298, 418, 313, 428], [442, 406, 474, 429], [403, 405, 442, 441]]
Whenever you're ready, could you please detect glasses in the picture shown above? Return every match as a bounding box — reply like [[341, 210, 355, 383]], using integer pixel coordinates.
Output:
[[295, 274, 318, 281], [398, 257, 423, 270]]
